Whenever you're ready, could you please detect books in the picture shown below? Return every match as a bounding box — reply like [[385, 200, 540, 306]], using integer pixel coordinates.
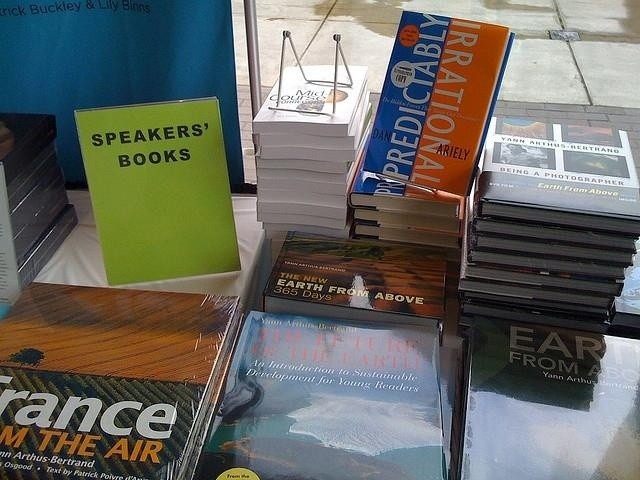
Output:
[[251, 9, 640, 347], [1, 113, 79, 301], [2, 282, 638, 480]]
